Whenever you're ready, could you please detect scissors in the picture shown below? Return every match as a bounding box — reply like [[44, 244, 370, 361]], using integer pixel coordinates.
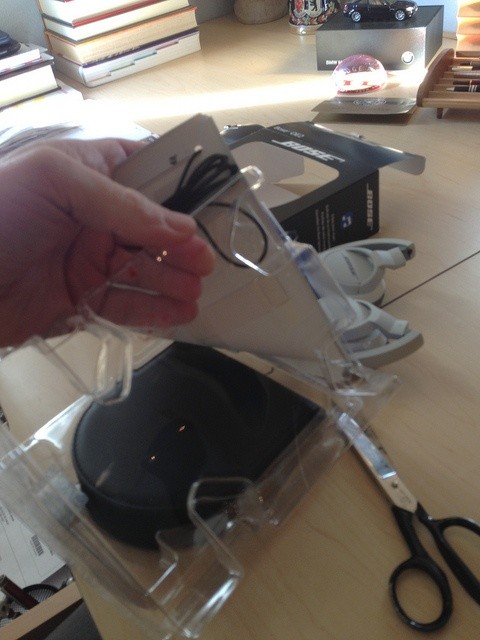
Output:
[[336, 411, 480, 632]]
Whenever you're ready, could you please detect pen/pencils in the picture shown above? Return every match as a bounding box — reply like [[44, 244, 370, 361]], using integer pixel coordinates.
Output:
[[452, 66, 480, 70], [453, 80, 480, 84], [461, 62, 480, 66], [446, 86, 479, 92], [453, 71, 480, 77]]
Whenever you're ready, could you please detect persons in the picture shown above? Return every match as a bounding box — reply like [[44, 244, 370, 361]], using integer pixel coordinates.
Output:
[[0, 137, 218, 351]]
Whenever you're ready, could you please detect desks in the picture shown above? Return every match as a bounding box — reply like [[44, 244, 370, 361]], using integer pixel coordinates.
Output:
[[1, 9, 479, 640]]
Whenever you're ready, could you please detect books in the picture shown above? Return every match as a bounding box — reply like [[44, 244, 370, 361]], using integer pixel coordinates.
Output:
[[0, 41, 41, 75], [40, 1, 199, 87], [0, 61, 59, 111]]
